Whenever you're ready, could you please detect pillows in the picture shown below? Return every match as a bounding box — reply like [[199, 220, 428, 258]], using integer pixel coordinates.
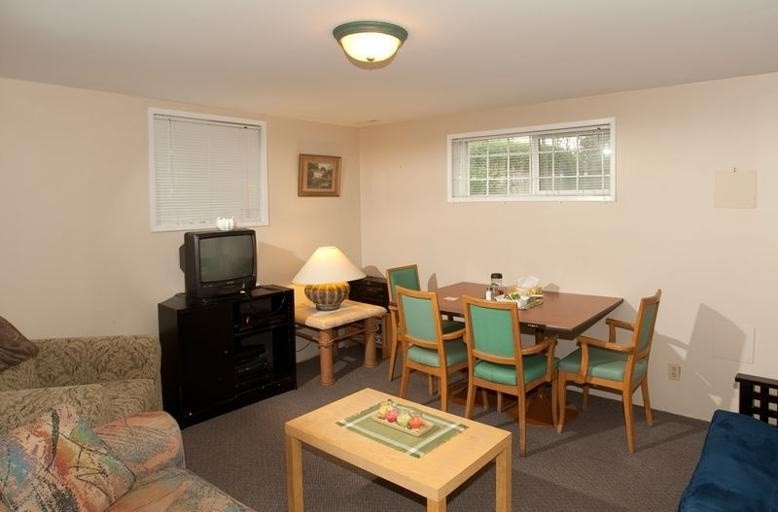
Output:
[[0, 402, 136, 512], [0, 316, 40, 372]]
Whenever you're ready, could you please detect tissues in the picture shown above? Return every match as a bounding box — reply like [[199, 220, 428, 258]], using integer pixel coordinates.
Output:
[[515, 276, 542, 297]]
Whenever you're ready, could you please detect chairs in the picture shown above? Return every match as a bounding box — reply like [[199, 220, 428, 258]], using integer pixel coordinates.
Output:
[[0, 335, 158, 432], [461, 293, 559, 459], [0, 410, 255, 512], [384, 263, 465, 381], [394, 284, 490, 418], [556, 289, 662, 454]]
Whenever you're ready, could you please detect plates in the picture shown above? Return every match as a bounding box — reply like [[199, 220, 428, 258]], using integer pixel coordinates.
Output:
[[372, 414, 434, 436]]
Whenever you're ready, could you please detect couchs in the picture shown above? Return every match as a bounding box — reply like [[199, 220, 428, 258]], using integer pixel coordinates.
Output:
[[676, 373, 778, 512]]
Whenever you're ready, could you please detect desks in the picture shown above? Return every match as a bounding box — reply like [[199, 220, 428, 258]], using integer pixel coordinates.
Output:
[[294, 299, 387, 385], [421, 281, 622, 427]]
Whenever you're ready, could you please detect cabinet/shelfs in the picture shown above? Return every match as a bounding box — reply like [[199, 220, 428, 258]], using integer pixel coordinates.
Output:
[[157, 285, 298, 430]]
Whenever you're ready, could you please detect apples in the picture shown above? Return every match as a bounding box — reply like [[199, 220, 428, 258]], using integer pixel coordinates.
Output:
[[386, 411, 397, 422], [407, 417, 422, 429]]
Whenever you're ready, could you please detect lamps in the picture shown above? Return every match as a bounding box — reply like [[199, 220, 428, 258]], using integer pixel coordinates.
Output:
[[292, 245, 366, 311], [332, 21, 408, 63]]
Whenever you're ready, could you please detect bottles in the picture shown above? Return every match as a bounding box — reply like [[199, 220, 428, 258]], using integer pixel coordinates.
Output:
[[485, 273, 503, 301]]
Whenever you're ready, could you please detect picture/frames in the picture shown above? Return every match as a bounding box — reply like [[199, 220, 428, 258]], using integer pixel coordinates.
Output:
[[298, 153, 343, 197]]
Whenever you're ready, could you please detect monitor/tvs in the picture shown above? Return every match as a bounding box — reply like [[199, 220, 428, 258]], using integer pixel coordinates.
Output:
[[179, 229, 257, 300]]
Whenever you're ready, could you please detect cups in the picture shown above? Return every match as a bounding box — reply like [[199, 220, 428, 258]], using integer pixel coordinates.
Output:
[[216, 216, 235, 230]]
